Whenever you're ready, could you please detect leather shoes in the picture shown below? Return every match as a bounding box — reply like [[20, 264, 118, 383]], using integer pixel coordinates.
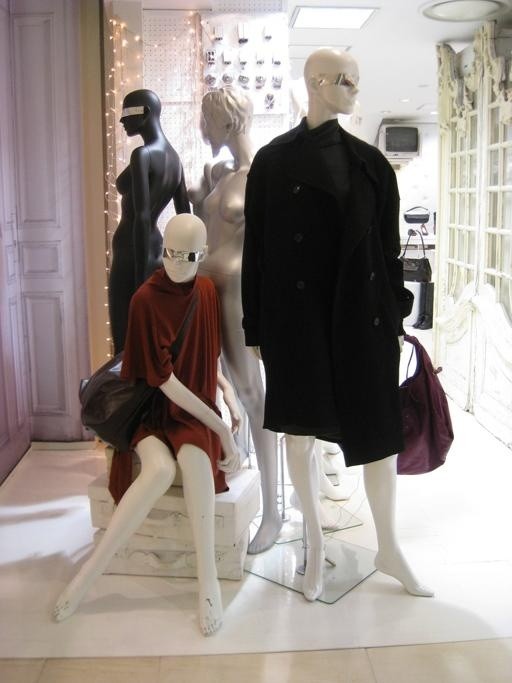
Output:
[[408, 225, 428, 236]]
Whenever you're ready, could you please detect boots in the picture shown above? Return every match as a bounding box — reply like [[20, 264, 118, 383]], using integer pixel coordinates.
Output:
[[413, 282, 434, 330]]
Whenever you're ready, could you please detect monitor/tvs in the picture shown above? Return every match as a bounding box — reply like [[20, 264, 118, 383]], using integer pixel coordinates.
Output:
[[379, 124, 421, 159]]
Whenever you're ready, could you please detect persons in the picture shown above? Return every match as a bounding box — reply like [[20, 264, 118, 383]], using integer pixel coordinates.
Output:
[[288, 438, 353, 529], [53, 213, 246, 636], [105, 88, 190, 358], [195, 87, 285, 555], [240, 52, 437, 603]]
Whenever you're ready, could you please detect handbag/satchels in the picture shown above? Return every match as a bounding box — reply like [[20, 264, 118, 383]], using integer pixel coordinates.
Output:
[[397, 336, 453, 475], [78, 372, 151, 451], [399, 256, 431, 282], [404, 206, 429, 223]]
